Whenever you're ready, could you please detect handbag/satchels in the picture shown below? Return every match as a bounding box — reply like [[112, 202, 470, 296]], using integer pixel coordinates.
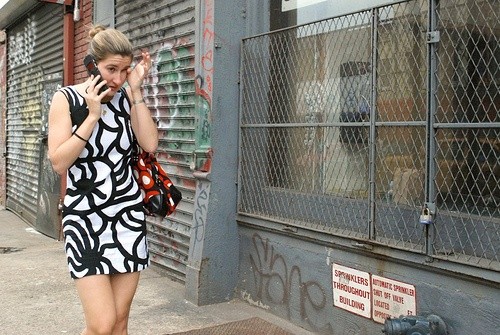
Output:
[[121, 86, 183, 218]]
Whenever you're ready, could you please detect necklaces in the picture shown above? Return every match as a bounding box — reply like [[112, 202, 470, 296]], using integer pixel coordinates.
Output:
[[101, 103, 108, 117]]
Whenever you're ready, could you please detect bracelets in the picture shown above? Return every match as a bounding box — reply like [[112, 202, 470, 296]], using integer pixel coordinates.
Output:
[[133, 99, 144, 104], [74, 132, 88, 143]]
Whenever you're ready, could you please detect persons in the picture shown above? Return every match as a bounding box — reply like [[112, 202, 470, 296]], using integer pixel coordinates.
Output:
[[46, 22, 159, 335]]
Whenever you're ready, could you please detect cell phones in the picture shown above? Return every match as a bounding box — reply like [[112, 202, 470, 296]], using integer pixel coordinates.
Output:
[[84, 54, 108, 94]]
